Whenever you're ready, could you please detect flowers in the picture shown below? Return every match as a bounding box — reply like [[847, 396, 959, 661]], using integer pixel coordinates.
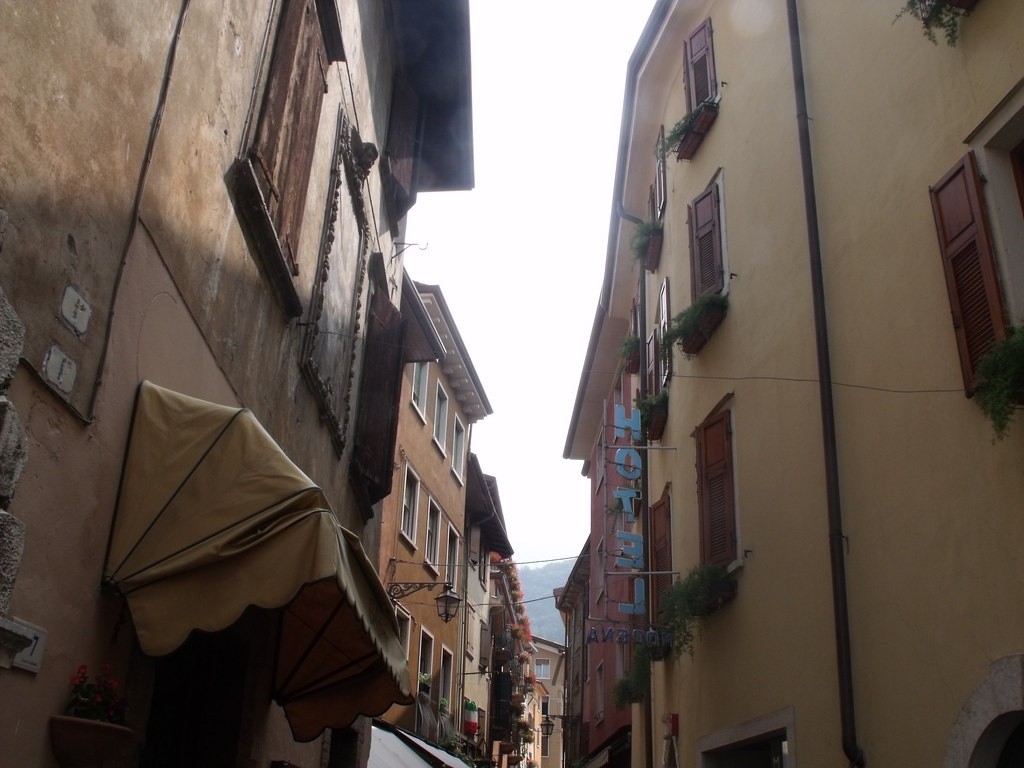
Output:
[[62, 658, 129, 726]]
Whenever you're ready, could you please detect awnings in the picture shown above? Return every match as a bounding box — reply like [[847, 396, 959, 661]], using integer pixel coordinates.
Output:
[[101, 379, 416, 744], [366, 718, 471, 768]]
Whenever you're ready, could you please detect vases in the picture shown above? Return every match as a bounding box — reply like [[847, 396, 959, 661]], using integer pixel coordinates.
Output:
[[49, 714, 138, 768]]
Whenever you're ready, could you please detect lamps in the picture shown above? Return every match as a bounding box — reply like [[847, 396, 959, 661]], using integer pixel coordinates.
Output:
[[540, 714, 581, 738], [387, 576, 463, 623]]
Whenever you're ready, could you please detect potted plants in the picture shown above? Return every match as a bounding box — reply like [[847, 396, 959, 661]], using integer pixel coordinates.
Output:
[[660, 293, 729, 361], [654, 118, 705, 171], [657, 561, 736, 664], [627, 220, 663, 275], [682, 104, 717, 134], [629, 390, 669, 442], [615, 335, 640, 376]]
